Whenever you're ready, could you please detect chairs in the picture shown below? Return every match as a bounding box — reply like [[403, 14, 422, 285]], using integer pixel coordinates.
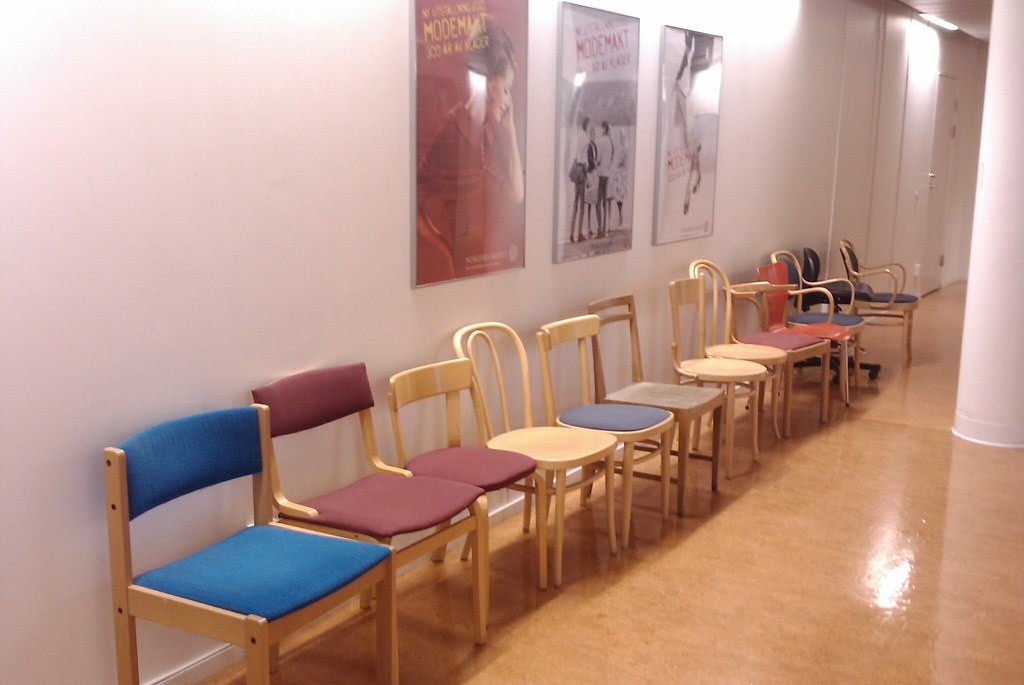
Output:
[[756, 247, 865, 413], [535, 313, 673, 554], [246, 362, 487, 672], [690, 259, 786, 455], [721, 280, 832, 441], [383, 357, 546, 627], [667, 272, 766, 479], [104, 402, 400, 685], [837, 237, 919, 370], [453, 321, 617, 587], [586, 294, 725, 519]]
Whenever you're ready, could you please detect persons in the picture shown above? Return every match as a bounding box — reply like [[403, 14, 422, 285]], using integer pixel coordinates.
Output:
[[417, 11, 527, 283], [672, 30, 714, 215], [569, 115, 629, 243]]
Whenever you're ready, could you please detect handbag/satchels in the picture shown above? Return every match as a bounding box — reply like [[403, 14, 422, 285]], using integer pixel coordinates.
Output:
[[582, 171, 600, 205]]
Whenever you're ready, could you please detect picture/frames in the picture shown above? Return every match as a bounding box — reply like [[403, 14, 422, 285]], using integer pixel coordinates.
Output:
[[552, 2, 640, 264], [652, 24, 724, 245], [406, 0, 531, 289]]
